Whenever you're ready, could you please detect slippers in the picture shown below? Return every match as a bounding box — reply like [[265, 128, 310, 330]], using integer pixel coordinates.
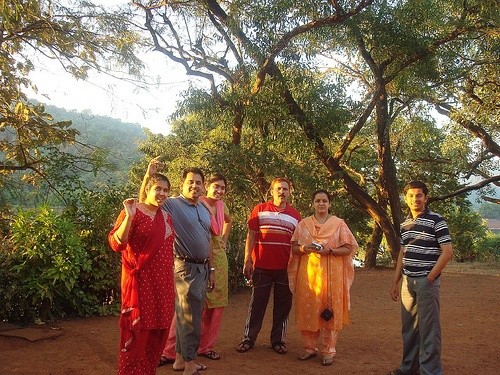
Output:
[[175, 361, 206, 371], [322, 357, 334, 366], [237, 343, 250, 352], [204, 350, 220, 359], [157, 356, 175, 366], [298, 351, 317, 360], [273, 344, 288, 353]]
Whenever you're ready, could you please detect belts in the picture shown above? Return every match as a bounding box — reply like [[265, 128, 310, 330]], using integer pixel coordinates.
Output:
[[173, 254, 210, 265]]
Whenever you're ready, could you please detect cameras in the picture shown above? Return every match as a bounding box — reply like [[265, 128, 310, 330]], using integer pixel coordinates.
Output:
[[312, 241, 323, 251]]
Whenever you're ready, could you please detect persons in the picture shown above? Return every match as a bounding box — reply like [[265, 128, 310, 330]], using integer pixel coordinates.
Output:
[[286, 189, 359, 365], [107, 174, 177, 375], [138, 156, 216, 375], [236, 177, 302, 355], [390, 181, 453, 375], [158, 173, 232, 366]]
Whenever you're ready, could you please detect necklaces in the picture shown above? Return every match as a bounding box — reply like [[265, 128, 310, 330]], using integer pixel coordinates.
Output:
[[323, 214, 329, 225]]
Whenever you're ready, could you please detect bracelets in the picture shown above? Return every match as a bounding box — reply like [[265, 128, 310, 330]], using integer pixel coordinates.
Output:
[[209, 268, 215, 271], [329, 248, 337, 258]]
